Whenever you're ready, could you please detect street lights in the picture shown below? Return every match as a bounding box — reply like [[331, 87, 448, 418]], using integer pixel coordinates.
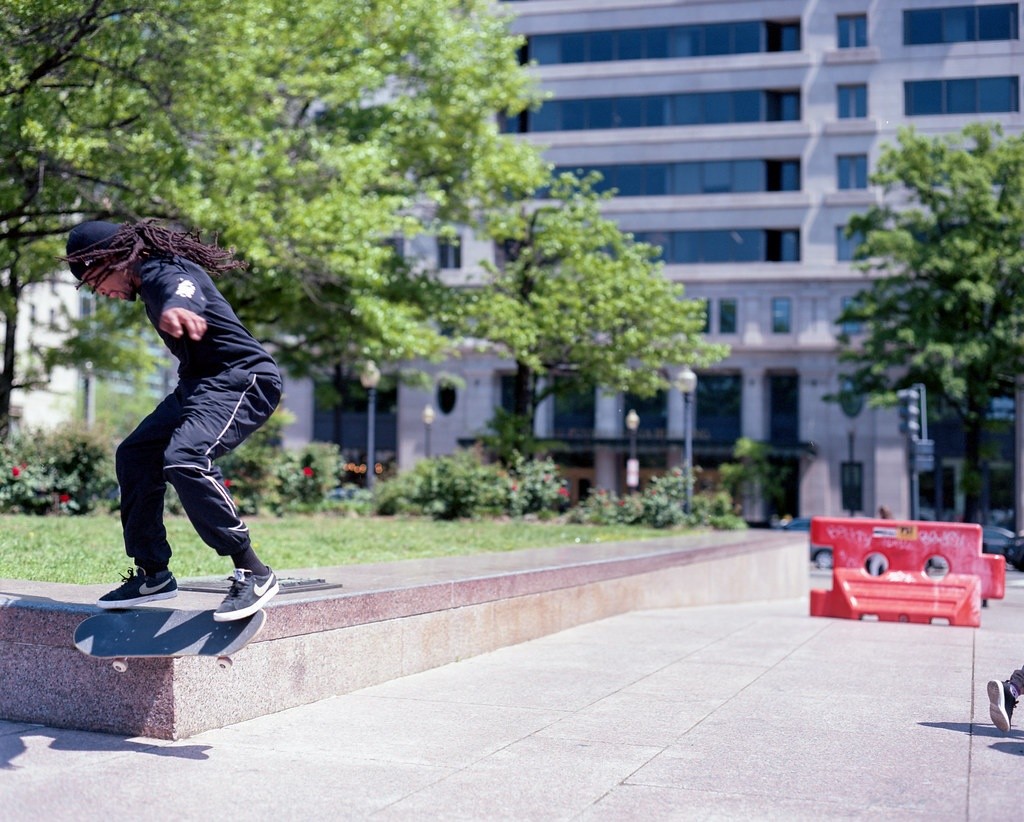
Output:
[[676, 363, 701, 521], [359, 359, 380, 490], [624, 409, 643, 495], [422, 402, 435, 462]]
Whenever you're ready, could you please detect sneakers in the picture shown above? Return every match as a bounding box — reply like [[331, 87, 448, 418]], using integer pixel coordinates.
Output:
[[97, 566, 178, 609], [212, 565, 279, 622]]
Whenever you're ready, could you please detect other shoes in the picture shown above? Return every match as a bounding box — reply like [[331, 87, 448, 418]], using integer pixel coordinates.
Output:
[[987, 679, 1018, 732]]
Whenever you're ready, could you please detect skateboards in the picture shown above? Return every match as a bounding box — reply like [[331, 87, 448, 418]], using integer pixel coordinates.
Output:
[[72, 608, 267, 672]]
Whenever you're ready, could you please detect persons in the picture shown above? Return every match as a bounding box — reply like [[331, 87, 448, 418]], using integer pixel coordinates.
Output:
[[986, 665, 1024, 733], [63, 215, 285, 621], [878, 505, 893, 519]]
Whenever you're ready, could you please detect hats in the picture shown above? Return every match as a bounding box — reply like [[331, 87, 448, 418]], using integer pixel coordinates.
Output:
[[65, 222, 122, 280]]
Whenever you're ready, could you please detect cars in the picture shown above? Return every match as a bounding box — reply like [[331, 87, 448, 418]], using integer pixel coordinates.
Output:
[[1005, 529, 1024, 570], [981, 525, 1016, 557]]
